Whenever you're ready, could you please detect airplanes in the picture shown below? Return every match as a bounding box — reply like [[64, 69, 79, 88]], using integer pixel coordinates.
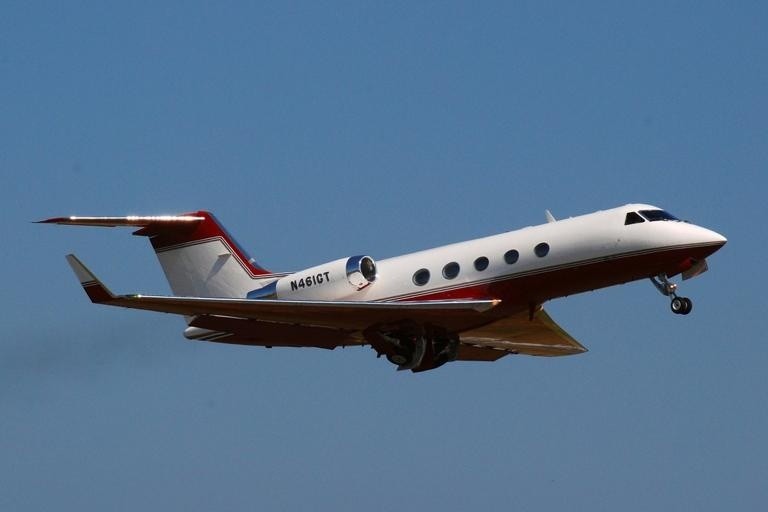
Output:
[[29, 202, 727, 375]]
